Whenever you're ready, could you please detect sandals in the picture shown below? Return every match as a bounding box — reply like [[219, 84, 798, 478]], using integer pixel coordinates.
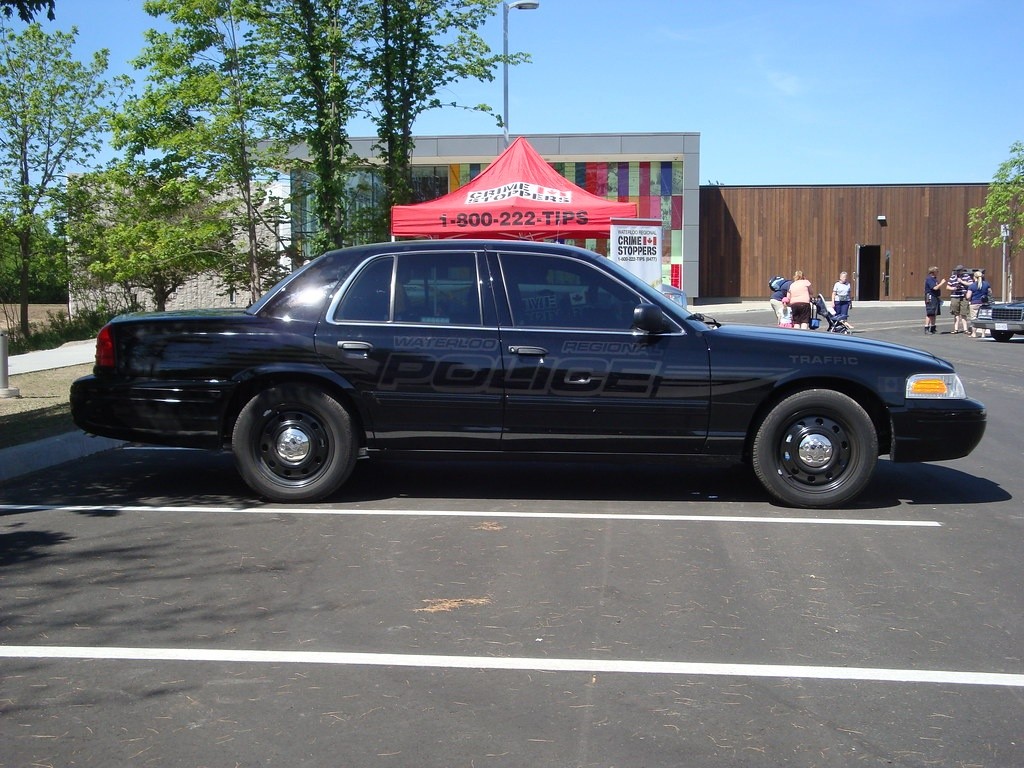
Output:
[[963, 329, 971, 335], [951, 330, 959, 333]]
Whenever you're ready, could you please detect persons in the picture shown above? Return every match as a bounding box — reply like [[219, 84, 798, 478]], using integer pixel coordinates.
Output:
[[946, 264, 993, 338], [924, 266, 947, 335], [768, 271, 814, 329], [831, 271, 852, 322]]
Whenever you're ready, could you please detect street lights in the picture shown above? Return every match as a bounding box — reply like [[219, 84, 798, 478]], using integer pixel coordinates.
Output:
[[501, 1, 540, 147]]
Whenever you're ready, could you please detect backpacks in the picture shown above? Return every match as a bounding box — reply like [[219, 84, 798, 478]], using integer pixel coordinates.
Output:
[[769, 276, 792, 291]]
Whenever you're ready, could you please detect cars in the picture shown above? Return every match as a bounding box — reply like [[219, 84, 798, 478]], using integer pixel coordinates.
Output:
[[69, 239, 991, 508], [971, 301, 1023, 344]]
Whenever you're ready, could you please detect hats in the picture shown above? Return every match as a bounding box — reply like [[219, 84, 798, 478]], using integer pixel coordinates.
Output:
[[952, 265, 964, 271], [782, 297, 790, 305]]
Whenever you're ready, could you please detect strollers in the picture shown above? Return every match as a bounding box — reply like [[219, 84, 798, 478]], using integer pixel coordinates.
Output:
[[809, 294, 852, 335]]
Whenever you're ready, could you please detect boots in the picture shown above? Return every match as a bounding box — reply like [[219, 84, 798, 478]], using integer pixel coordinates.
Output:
[[925, 325, 932, 335], [930, 325, 938, 334]]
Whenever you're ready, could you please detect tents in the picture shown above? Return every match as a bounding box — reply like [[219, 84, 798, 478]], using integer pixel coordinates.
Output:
[[391, 136, 637, 243]]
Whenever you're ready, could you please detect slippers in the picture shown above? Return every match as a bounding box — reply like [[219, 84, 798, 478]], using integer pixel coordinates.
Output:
[[981, 334, 986, 338], [969, 335, 978, 338]]
